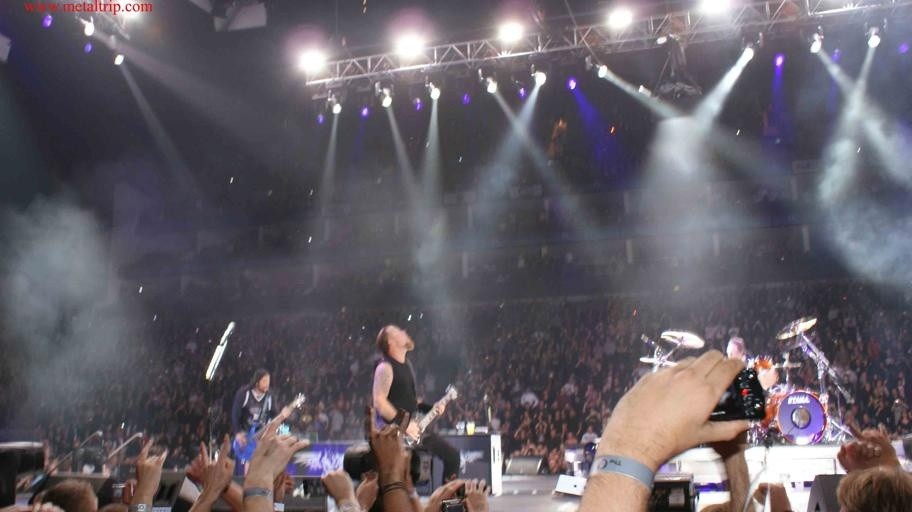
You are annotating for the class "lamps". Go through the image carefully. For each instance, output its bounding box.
[315,4,909,123]
[40,2,130,69]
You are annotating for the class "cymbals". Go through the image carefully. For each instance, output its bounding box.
[641,357,673,366]
[662,331,704,350]
[776,316,817,340]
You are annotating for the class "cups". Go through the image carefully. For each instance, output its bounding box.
[457,422,466,435]
[467,420,475,435]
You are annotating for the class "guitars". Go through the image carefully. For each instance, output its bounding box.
[233,393,306,459]
[402,385,458,449]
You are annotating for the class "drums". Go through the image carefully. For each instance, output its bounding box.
[755,356,779,389]
[762,391,827,445]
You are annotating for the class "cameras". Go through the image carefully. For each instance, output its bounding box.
[456,483,492,499]
[708,368,766,421]
